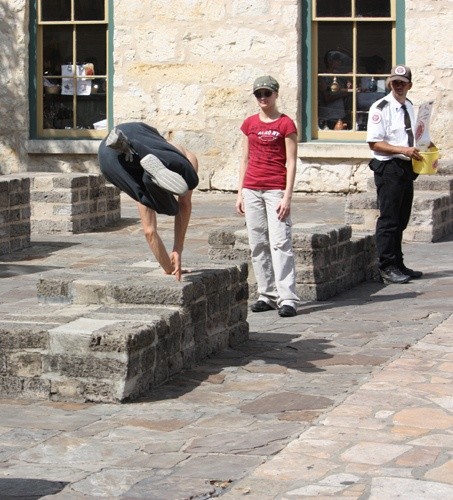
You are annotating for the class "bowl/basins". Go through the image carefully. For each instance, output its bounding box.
[45,85,59,94]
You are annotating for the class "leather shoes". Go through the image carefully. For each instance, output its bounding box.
[380,267,411,284]
[279,305,297,316]
[251,301,274,312]
[399,266,423,279]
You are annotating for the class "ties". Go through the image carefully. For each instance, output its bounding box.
[402,105,413,147]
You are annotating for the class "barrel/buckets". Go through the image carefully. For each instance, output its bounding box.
[412,147,439,175]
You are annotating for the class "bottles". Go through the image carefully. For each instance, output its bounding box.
[369,77,377,92]
[345,79,352,89]
[330,77,339,92]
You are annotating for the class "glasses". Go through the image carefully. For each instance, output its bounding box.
[254,91,277,98]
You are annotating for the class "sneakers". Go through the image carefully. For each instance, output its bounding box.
[105,127,139,162]
[140,154,189,194]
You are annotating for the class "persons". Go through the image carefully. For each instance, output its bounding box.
[366,64,442,286]
[98,123,199,282]
[236,77,298,317]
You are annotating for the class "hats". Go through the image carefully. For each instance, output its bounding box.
[253,75,279,93]
[389,65,411,83]
